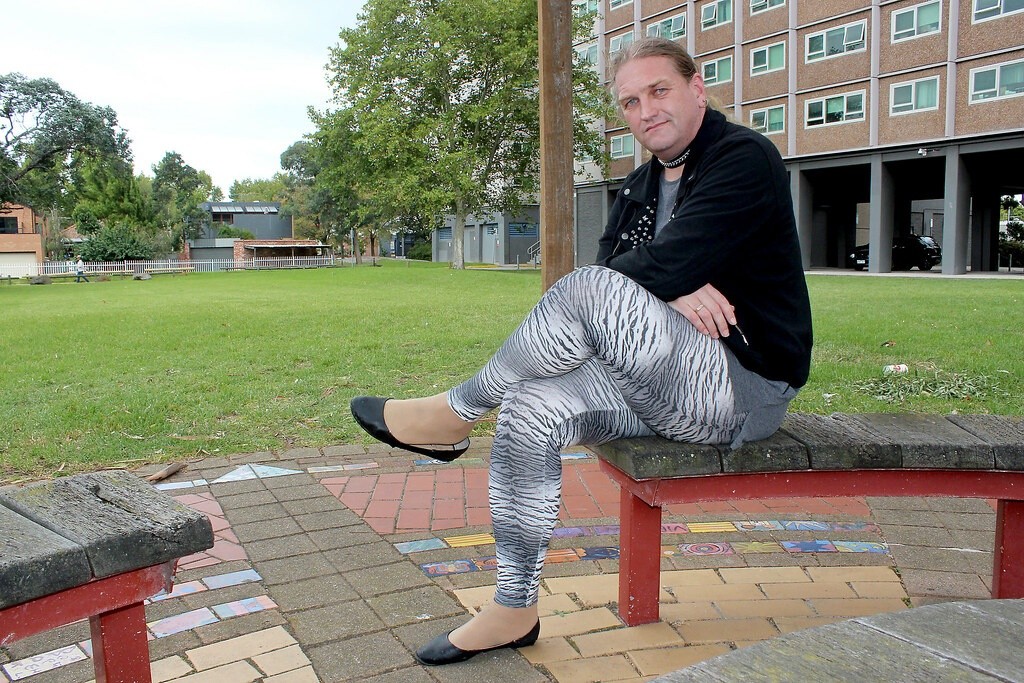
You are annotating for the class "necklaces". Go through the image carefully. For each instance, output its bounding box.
[658,143,691,169]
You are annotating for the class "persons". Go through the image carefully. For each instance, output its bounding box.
[351,38,814,665]
[75,255,89,283]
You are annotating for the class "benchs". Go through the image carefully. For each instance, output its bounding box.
[0,468,214,683]
[583,414,1024,624]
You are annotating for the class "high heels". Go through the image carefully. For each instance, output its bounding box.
[413,610,540,666]
[351,395,470,463]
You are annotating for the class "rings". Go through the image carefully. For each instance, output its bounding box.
[694,304,704,312]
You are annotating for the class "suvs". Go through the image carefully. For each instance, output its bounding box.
[849,235,943,272]
